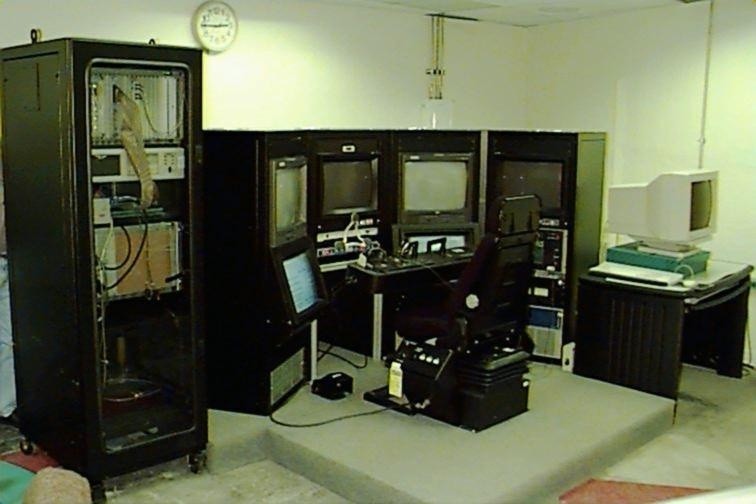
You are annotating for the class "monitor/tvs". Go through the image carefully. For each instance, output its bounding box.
[272,237,330,325]
[494,151,567,218]
[397,152,474,227]
[317,151,383,221]
[269,155,308,248]
[607,169,720,252]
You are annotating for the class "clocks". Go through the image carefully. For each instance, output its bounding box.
[192,2,237,56]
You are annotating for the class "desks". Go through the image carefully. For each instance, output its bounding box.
[573,262,755,422]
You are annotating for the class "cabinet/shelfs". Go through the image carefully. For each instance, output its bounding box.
[1,36,211,504]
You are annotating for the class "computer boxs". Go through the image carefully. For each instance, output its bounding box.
[606,241,710,274]
